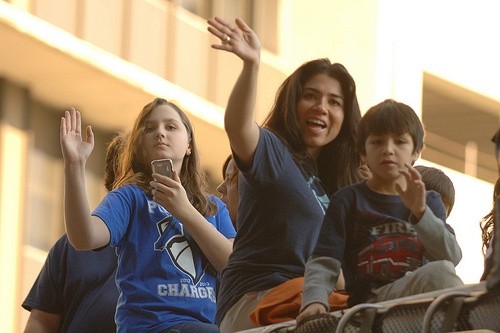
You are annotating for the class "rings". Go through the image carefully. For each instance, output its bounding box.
[222,36,230,44]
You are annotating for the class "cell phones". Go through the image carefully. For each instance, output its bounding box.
[151,159,173,183]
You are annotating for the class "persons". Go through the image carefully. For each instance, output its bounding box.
[60,98,237,333]
[206,15,362,333]
[217,153,237,228]
[20,134,131,333]
[296,95,500,325]
